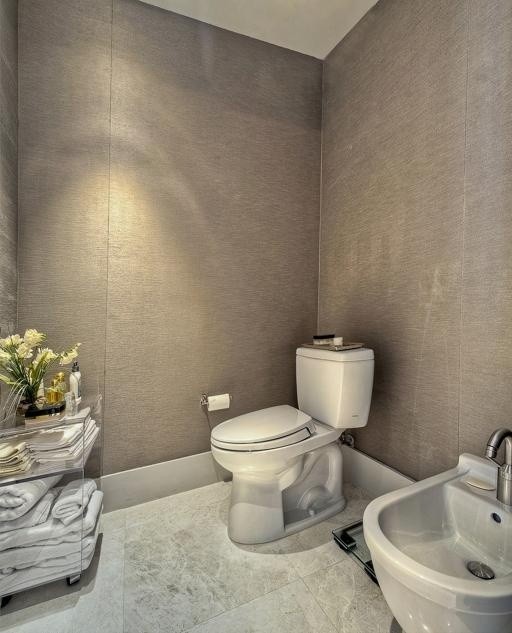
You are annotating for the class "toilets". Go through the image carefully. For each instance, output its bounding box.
[211,338,375,545]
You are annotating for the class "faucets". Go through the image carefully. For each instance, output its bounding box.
[485,429,512,505]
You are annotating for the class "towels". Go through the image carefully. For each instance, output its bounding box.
[1,419,104,601]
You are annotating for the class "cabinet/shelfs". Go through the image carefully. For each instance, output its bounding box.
[0,393,104,611]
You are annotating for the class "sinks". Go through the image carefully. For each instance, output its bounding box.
[363,451,512,633]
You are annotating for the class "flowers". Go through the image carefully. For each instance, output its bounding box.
[0,316,83,431]
